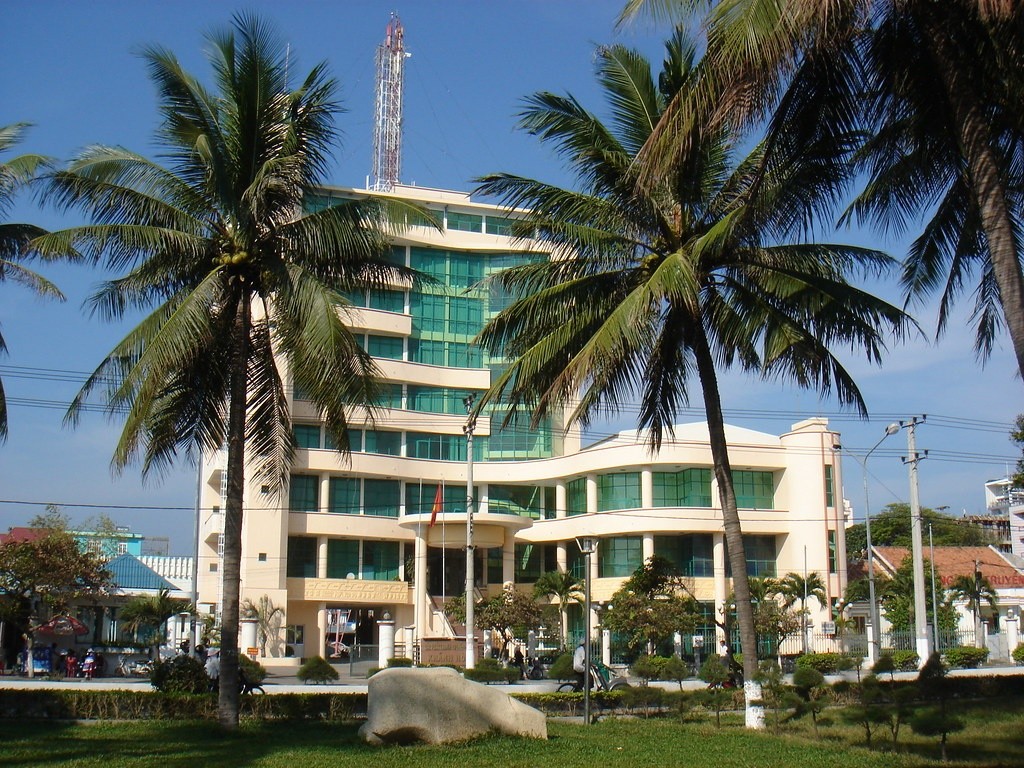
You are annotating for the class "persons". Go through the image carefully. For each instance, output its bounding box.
[514,647,527,677]
[204,648,220,691]
[47,642,64,657]
[574,639,594,691]
[0,640,8,674]
[177,642,189,656]
[720,641,728,657]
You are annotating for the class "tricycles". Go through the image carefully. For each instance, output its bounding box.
[556,660,632,693]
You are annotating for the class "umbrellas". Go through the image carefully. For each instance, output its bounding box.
[38,615,89,635]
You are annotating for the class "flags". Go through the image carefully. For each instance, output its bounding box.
[430,486,440,527]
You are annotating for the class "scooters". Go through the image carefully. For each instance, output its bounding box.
[66,651,99,681]
[114,654,155,679]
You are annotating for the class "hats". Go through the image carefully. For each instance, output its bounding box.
[580,639,585,644]
[207,647,220,656]
[87,648,94,652]
[180,642,188,648]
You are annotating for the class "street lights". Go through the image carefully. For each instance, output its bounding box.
[834,598,853,658]
[861,422,900,667]
[460,389,478,670]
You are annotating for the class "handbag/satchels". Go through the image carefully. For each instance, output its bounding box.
[596,663,610,682]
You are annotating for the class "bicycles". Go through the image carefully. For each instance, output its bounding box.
[211,677,267,696]
[513,658,544,680]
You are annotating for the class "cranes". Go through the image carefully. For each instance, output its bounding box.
[372,12,411,189]
